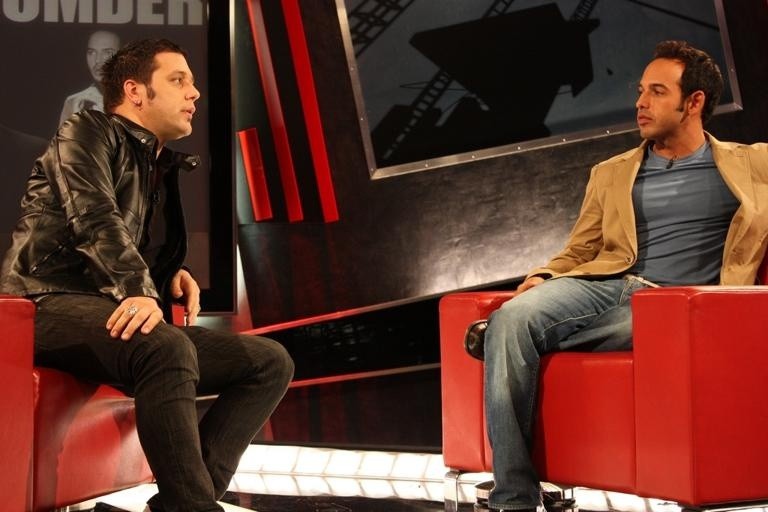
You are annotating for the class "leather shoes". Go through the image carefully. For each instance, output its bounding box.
[463,318,488,361]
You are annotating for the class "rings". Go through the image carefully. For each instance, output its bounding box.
[127,307,138,314]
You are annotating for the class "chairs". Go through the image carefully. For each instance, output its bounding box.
[0,293,186,512]
[437,229,767,509]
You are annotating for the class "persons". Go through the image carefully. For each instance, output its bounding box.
[478,38,768,512]
[0,41,298,512]
[56,26,123,126]
